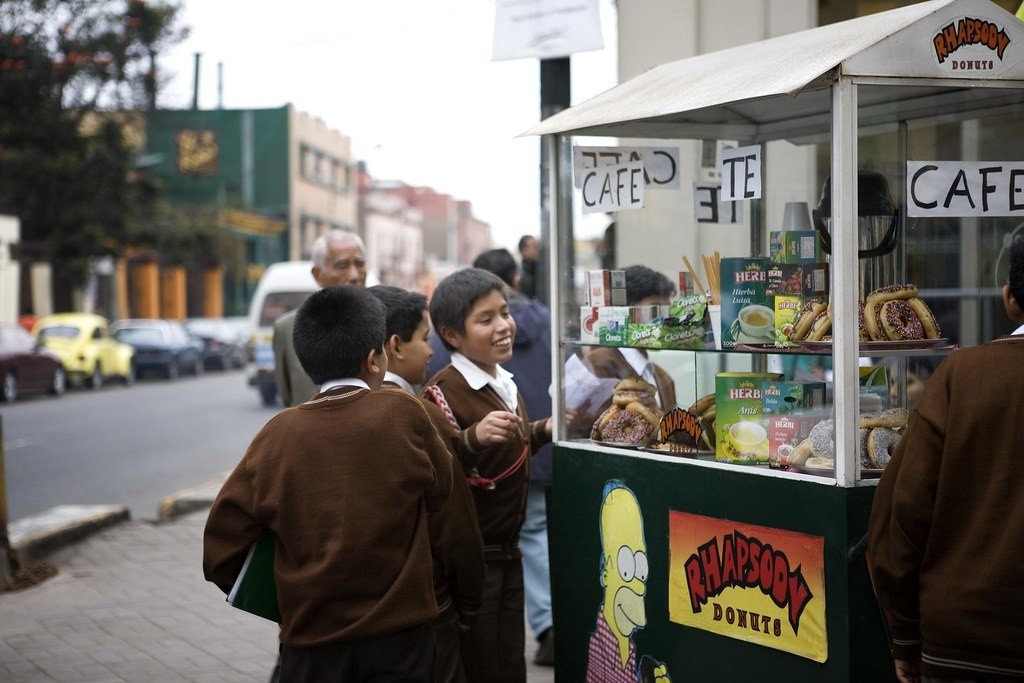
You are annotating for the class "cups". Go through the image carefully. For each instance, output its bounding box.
[781,202,811,231]
[738,304,775,340]
[730,422,767,450]
[708,304,722,350]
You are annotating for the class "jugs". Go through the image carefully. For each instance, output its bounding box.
[811,171,901,297]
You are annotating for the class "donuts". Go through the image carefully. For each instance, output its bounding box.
[787,407,911,469]
[588,378,717,453]
[787,283,942,342]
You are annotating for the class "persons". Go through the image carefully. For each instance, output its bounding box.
[201,287,486,683]
[426,247,556,672]
[547,265,678,443]
[417,265,579,683]
[516,235,542,298]
[271,226,367,408]
[865,237,1024,683]
[366,286,469,683]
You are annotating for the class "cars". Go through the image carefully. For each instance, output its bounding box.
[0,319,65,402]
[108,317,205,379]
[241,258,379,403]
[30,309,137,388]
[185,313,252,369]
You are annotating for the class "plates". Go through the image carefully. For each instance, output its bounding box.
[589,439,640,447]
[792,337,950,349]
[721,433,769,461]
[796,466,885,479]
[730,318,775,343]
[646,448,715,455]
